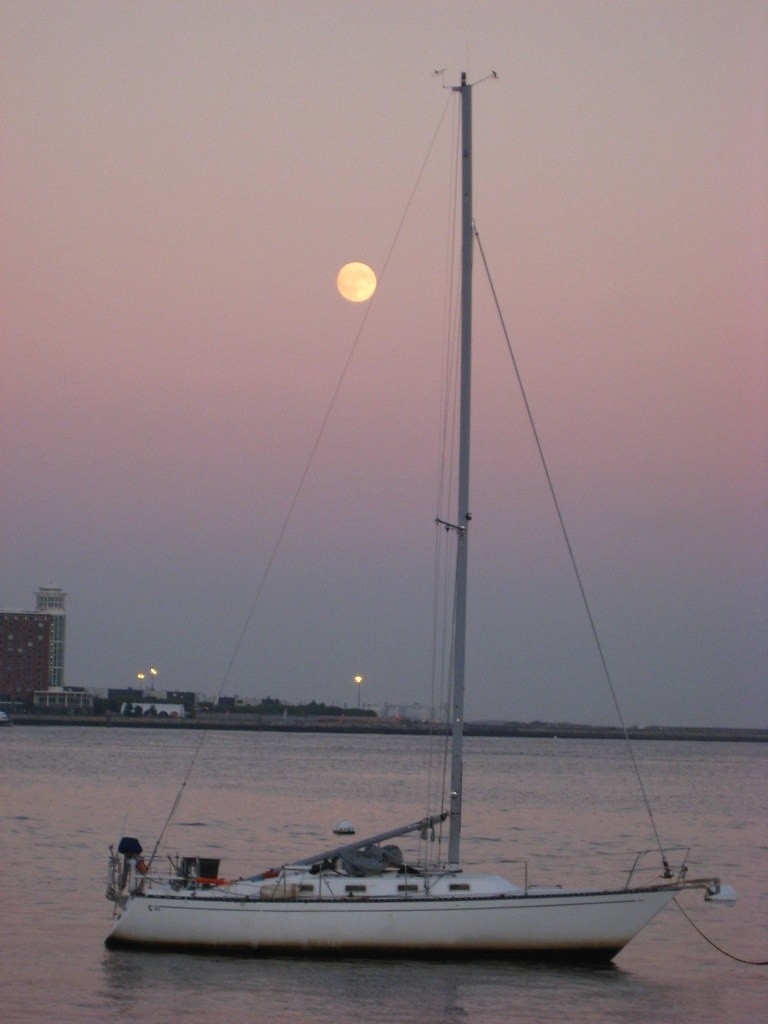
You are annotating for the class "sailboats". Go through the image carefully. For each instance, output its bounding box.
[92,69,722,960]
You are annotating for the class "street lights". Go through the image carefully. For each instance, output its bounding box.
[136,673,145,690]
[150,668,157,690]
[355,675,362,709]
